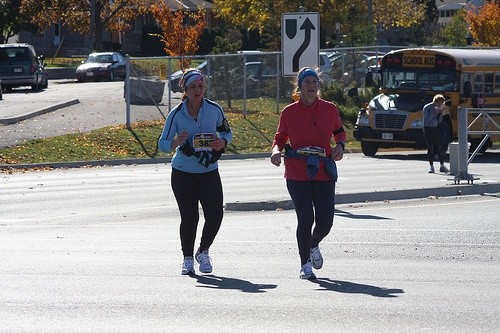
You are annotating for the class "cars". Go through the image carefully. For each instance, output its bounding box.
[0,43,48,92]
[76,52,132,82]
[168,51,386,100]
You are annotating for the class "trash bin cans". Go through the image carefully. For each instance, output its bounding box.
[450,142,471,174]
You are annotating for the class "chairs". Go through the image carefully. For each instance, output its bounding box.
[0,50,12,63]
[15,51,25,61]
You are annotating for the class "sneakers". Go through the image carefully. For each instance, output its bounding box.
[195,247,212,273]
[182,256,195,275]
[310,244,323,269]
[300,258,316,279]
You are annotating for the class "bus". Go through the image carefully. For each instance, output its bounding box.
[353,47,500,158]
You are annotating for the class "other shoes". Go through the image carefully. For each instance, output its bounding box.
[440,166,449,173]
[429,167,434,173]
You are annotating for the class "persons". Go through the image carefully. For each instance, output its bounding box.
[422,94,449,173]
[157,68,232,274]
[270,68,347,279]
